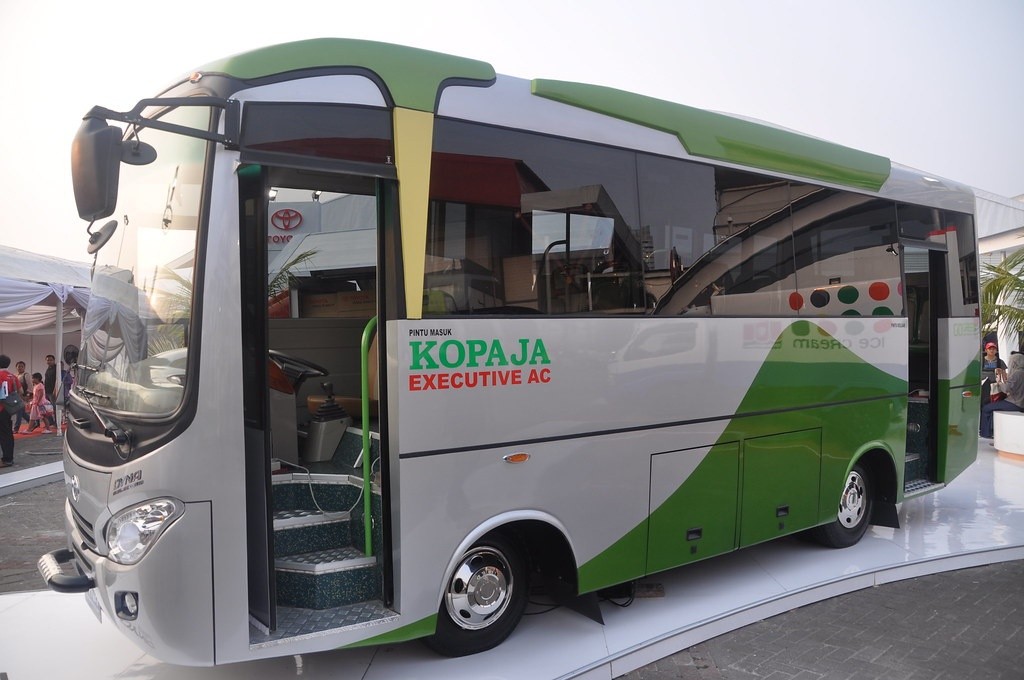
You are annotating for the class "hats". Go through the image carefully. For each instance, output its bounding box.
[985,342,997,349]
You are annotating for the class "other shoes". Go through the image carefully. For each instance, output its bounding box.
[0,458,14,468]
[21,430,31,435]
[41,430,52,434]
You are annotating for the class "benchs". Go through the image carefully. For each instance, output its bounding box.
[993,411,1024,462]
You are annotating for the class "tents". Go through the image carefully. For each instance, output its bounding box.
[0,245,139,437]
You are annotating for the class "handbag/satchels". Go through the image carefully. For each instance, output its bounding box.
[2,391,25,416]
[21,373,29,393]
[990,383,1007,403]
[37,403,54,416]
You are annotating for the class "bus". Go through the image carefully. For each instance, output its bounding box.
[34,37,980,664]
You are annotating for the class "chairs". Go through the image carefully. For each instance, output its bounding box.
[907,285,929,353]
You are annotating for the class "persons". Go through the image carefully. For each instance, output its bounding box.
[981,343,1008,404]
[0,354,23,467]
[43,355,56,428]
[979,353,1024,438]
[20,373,53,434]
[12,361,38,434]
[61,361,73,399]
